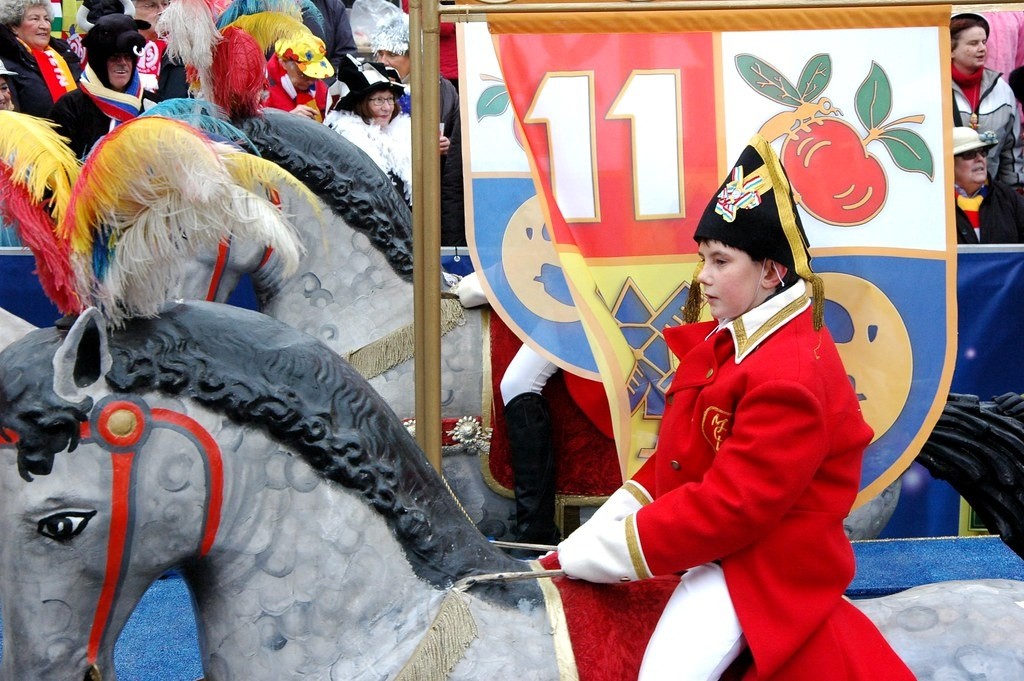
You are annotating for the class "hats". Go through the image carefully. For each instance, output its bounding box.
[953,126,998,156]
[81,13,151,88]
[0,59,19,75]
[232,11,335,79]
[683,134,826,331]
[338,53,390,103]
[950,12,990,38]
[214,25,269,115]
[385,66,406,99]
[350,0,410,53]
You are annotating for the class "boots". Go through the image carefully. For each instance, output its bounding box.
[496,392,562,556]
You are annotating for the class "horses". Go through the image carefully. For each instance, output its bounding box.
[96,105,1020,551]
[0,299,1020,681]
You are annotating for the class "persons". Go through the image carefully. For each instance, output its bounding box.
[558,133,917,681]
[949,12,1024,244]
[0,0,469,250]
[457,268,562,554]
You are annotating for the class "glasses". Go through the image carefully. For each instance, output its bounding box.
[107,53,134,63]
[136,2,170,10]
[956,149,989,160]
[368,95,396,106]
